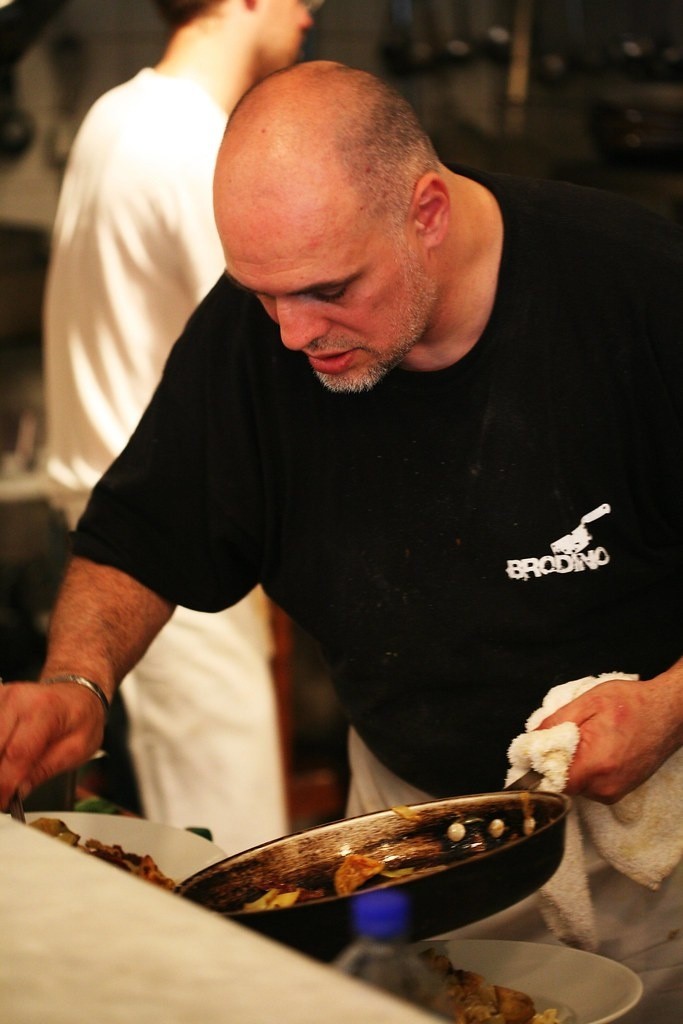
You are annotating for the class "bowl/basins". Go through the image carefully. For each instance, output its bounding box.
[3,811,228,885]
[403,940,643,1024]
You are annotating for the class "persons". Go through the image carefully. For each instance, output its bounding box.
[0,59,683,1023]
[42,0,316,858]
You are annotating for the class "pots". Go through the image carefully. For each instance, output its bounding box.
[172,770,572,966]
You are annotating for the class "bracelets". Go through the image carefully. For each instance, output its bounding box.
[42,676,110,723]
